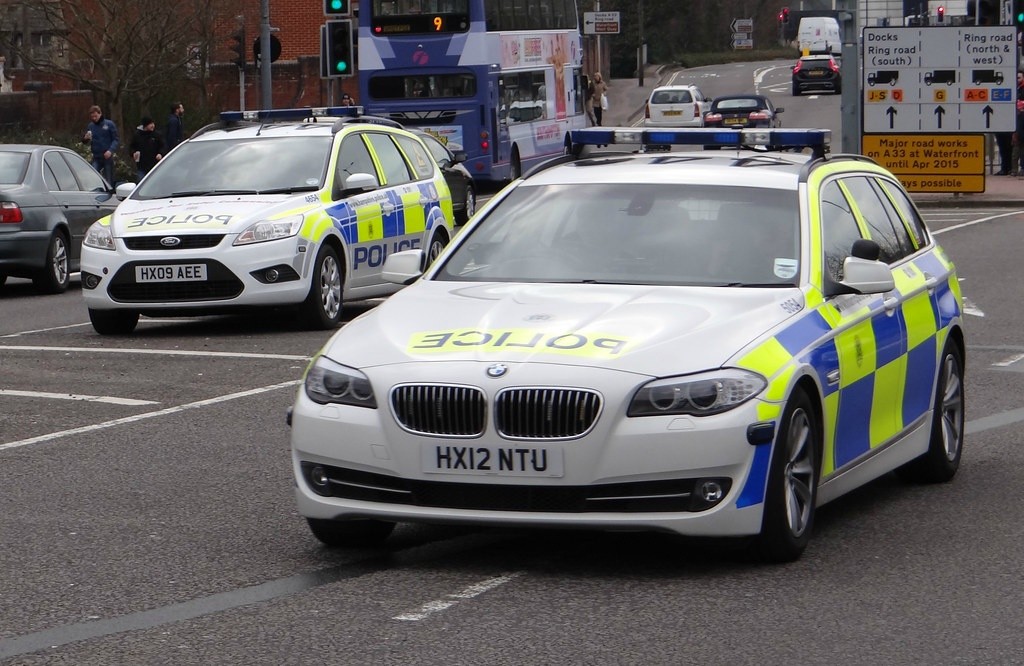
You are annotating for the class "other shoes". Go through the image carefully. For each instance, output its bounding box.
[994,169,1008,175]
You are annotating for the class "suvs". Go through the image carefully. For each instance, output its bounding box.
[645,82,712,128]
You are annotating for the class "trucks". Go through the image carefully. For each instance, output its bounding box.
[796,16,841,55]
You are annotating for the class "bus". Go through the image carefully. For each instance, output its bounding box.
[354,0,586,194]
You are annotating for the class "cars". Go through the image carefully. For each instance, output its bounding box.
[79,106,476,336]
[703,94,784,150]
[285,128,966,564]
[790,55,841,97]
[0,143,128,294]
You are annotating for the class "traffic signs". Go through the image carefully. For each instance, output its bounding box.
[862,25,1017,134]
[583,11,620,35]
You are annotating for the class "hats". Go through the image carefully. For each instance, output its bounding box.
[343,94,350,99]
[142,116,154,128]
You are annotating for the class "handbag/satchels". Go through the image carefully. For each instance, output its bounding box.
[600,93,608,110]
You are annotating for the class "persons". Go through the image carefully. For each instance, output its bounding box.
[167,102,184,153]
[584,72,608,126]
[81,105,120,189]
[340,93,357,106]
[993,71,1024,177]
[130,116,164,182]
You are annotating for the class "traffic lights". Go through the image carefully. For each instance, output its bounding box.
[1011,0,1024,31]
[778,8,789,23]
[323,0,351,17]
[326,19,353,80]
[231,28,245,70]
[938,7,943,22]
